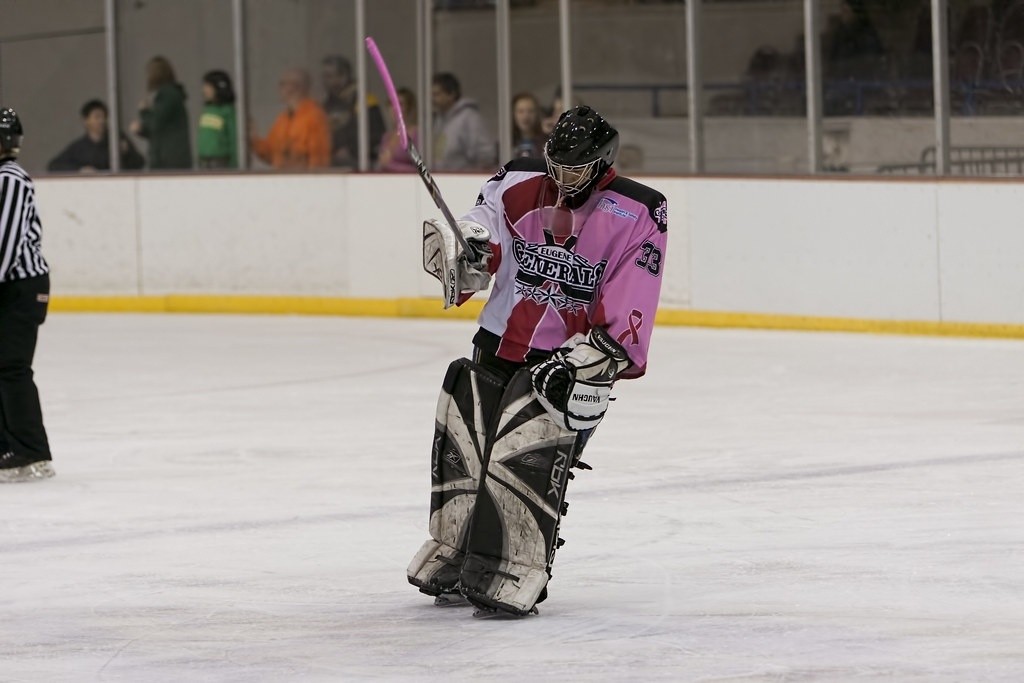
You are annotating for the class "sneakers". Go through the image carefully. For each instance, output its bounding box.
[0,450,57,482]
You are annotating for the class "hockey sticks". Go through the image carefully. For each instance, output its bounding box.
[363,33,487,273]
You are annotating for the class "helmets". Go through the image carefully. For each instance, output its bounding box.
[543,105,620,209]
[0,108,22,133]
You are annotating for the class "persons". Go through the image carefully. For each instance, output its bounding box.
[406,105,668,618]
[248,54,419,173]
[0,109,52,469]
[49,55,238,174]
[431,71,577,171]
